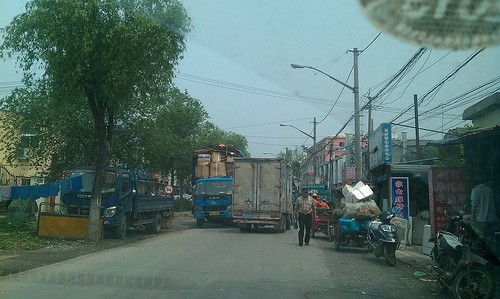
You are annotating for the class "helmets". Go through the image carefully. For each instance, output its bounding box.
[311,193,318,197]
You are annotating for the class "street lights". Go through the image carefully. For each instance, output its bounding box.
[279,123,316,186]
[291,63,363,183]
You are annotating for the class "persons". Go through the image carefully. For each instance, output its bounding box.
[319,199,328,208]
[312,192,320,204]
[294,187,319,247]
[470,178,500,231]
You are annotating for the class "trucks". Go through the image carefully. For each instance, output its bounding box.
[61,166,175,240]
[231,157,294,234]
[190,144,246,228]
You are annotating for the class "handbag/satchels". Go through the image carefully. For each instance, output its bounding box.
[313,220,319,232]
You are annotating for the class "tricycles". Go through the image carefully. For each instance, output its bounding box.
[310,199,372,250]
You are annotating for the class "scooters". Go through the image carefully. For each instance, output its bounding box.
[363,210,401,266]
[427,208,499,299]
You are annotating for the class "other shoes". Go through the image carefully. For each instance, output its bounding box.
[299,242,303,247]
[305,243,309,245]
